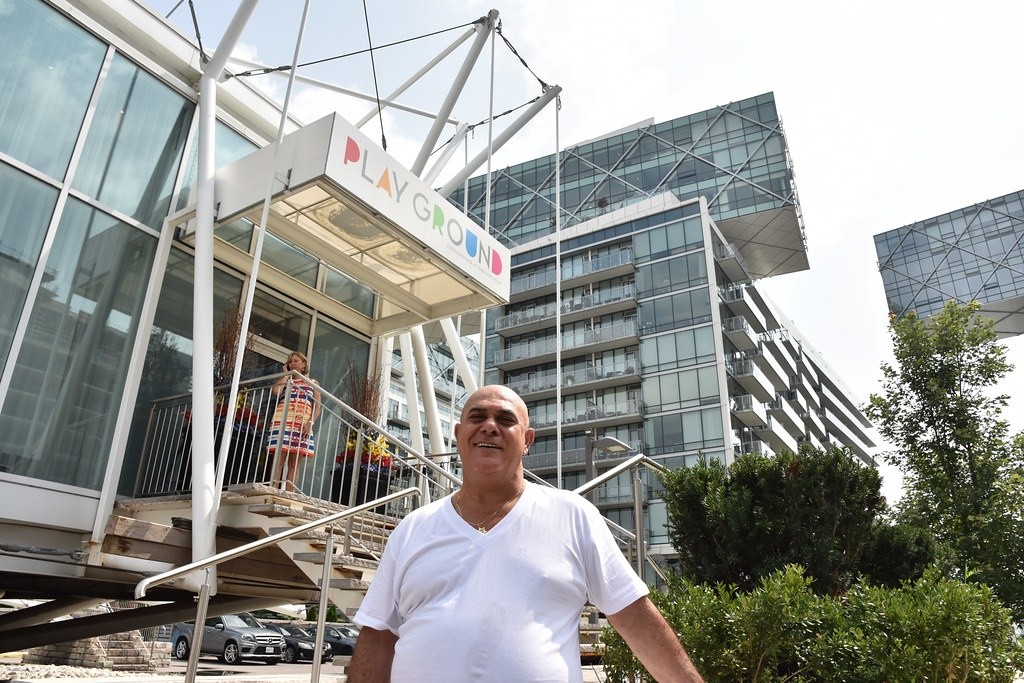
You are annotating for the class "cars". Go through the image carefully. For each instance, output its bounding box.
[170,615,287,666]
[262,622,332,664]
[305,626,357,656]
[330,627,360,638]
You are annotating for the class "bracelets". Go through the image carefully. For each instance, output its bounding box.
[309,421,314,425]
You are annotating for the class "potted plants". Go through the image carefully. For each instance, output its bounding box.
[565,375,575,386]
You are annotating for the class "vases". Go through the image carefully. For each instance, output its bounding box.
[178,432,258,493]
[328,468,395,515]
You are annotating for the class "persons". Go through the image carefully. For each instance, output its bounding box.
[266,352,321,493]
[346,384,704,683]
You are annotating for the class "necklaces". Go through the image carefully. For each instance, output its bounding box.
[456,491,520,534]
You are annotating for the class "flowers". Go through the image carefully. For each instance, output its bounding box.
[334,356,400,476]
[176,298,277,435]
